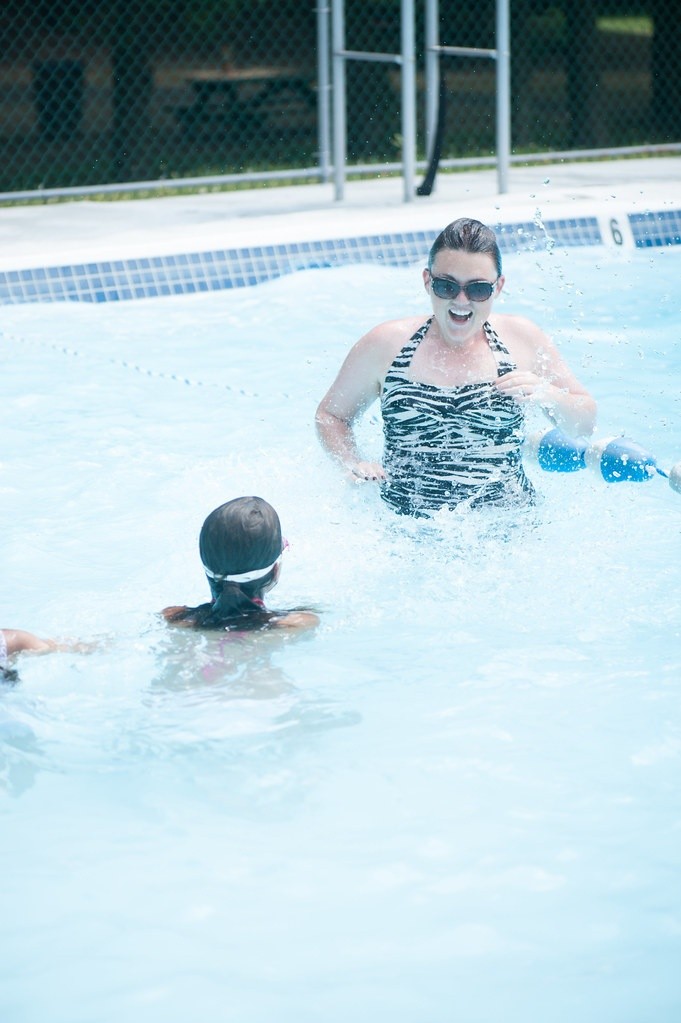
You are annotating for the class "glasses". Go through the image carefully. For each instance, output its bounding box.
[280,536,291,556]
[430,275,498,302]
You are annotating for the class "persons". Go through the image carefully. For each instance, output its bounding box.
[0,492,318,652]
[313,218,593,515]
